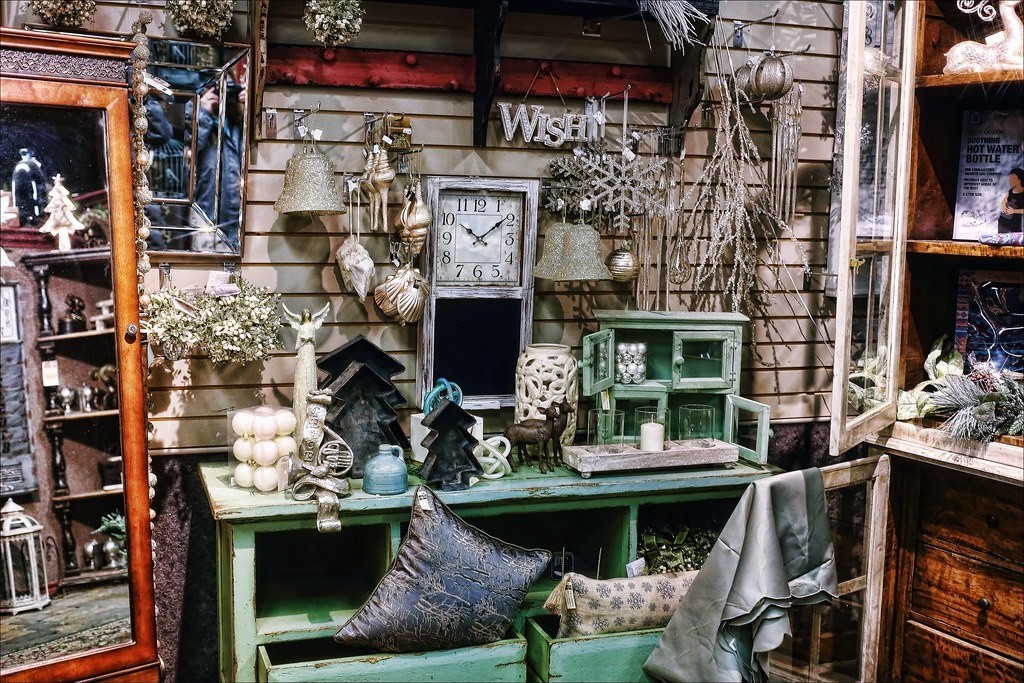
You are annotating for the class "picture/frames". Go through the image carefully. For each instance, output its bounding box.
[20,21,254,264]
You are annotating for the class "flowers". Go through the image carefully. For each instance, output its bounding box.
[165,0,235,42]
[137,275,287,365]
[20,0,97,28]
[302,0,367,50]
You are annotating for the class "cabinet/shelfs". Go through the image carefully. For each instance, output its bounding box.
[582,310,770,466]
[752,0,1024,683]
[22,247,130,590]
[198,439,787,683]
[0,48,161,683]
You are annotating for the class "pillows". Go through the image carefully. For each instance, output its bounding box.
[334,480,555,653]
[544,571,701,640]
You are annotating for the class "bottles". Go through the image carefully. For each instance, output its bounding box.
[12,147,47,229]
[362,444,407,495]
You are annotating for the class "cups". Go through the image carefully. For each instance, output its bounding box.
[587,409,625,455]
[679,404,714,448]
[634,406,672,452]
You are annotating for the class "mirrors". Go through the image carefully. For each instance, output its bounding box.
[0,102,140,675]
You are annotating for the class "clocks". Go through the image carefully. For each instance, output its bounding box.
[425,175,540,299]
